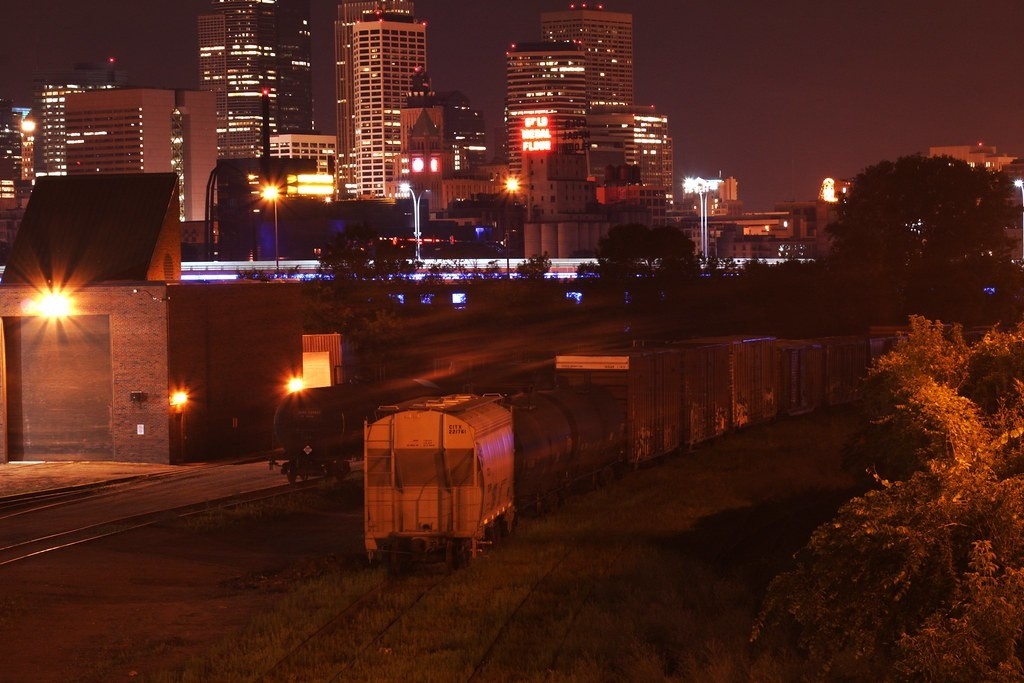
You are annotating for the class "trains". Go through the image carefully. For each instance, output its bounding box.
[363,320,1005,575]
[272,368,388,491]
